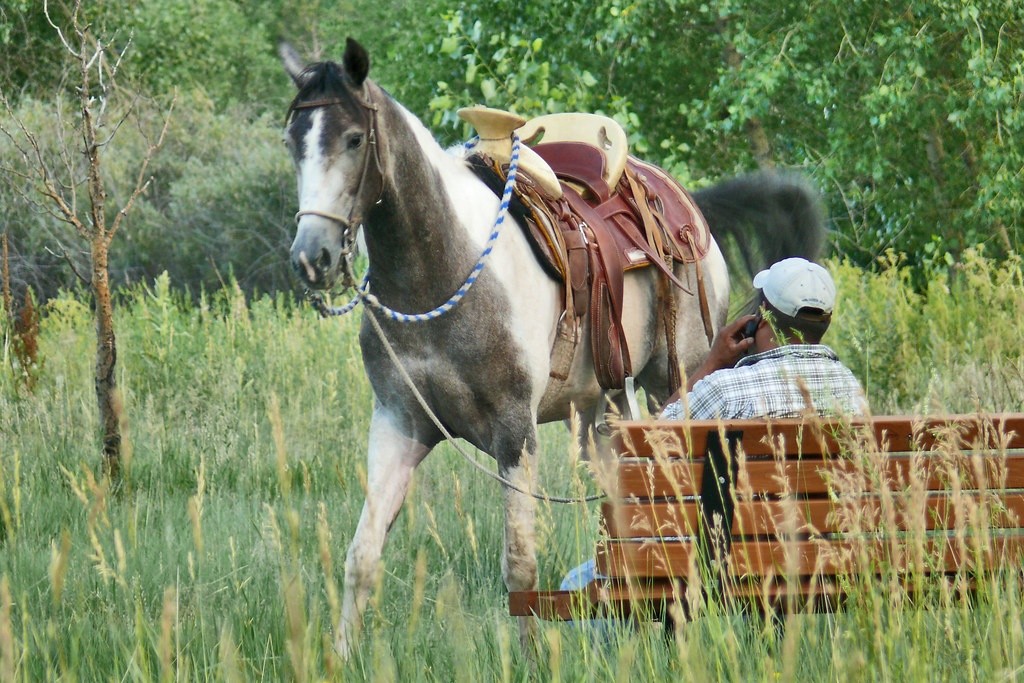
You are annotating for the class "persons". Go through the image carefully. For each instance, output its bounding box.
[558,257,874,592]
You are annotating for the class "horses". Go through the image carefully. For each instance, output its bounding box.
[277,36,825,660]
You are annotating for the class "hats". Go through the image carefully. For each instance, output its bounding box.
[753,257,836,320]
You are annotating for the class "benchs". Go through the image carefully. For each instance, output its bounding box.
[508,413,1024,648]
